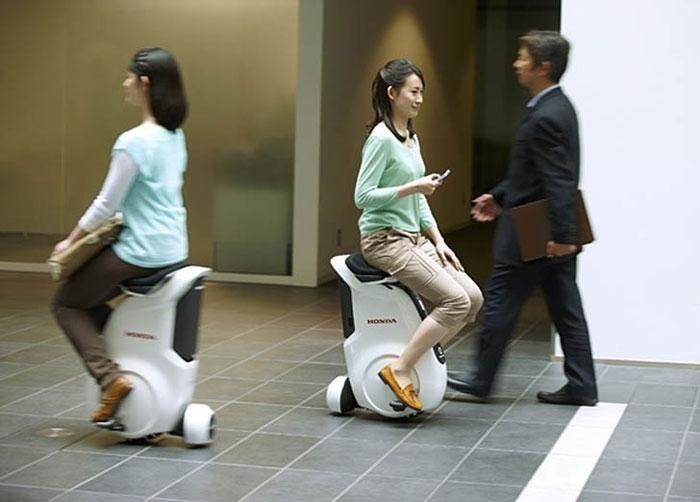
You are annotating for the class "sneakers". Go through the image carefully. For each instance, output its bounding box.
[377,363,424,413]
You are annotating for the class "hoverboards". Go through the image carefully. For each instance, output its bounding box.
[326,254,448,423]
[91,265,215,449]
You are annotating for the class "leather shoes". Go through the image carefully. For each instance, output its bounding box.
[536,387,599,406]
[447,375,489,399]
[88,376,133,422]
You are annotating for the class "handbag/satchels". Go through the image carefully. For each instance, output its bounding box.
[45,216,125,284]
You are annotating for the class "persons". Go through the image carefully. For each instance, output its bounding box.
[353,57,484,411]
[447,28,599,406]
[49,47,190,424]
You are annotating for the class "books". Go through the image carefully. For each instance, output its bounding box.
[510,189,594,264]
